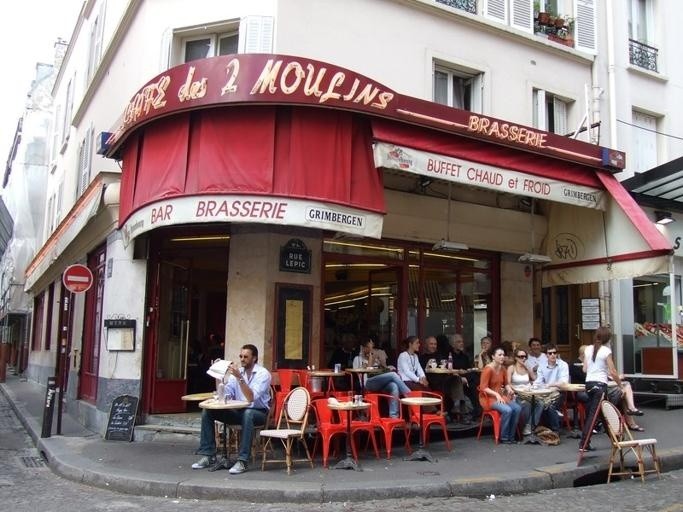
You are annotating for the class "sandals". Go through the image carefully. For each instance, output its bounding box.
[628,423,638,430]
[625,406,643,417]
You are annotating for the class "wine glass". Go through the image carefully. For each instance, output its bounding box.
[442,359,447,367]
[212,391,219,401]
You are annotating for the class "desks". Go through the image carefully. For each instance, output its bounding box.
[556,382,586,432]
[425,367,481,424]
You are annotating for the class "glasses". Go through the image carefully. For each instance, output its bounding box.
[238,354,247,359]
[547,351,556,355]
[516,355,526,359]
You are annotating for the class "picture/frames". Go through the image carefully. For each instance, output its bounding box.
[272,282,314,371]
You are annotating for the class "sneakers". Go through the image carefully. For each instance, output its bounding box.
[501,424,532,444]
[228,461,248,475]
[579,443,594,452]
[191,456,217,469]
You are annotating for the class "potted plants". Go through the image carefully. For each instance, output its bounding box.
[534,1,575,39]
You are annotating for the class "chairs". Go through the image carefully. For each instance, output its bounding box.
[180,368,452,475]
[476,385,521,446]
[600,400,662,484]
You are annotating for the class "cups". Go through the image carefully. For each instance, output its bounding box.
[334,364,342,373]
[224,394,232,405]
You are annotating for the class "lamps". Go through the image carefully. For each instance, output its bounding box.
[654,210,675,225]
[518,197,552,263]
[432,181,469,254]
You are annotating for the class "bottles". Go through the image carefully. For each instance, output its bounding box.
[368,352,374,367]
[355,395,362,405]
[432,359,437,369]
[448,355,453,371]
[358,345,365,369]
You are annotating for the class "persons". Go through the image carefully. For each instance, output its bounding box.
[419,327,645,453]
[192,344,272,474]
[327,334,361,395]
[353,335,412,428]
[397,336,449,416]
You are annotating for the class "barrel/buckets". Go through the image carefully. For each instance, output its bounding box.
[307,376,325,393]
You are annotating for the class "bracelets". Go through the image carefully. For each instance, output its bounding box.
[369,352,374,355]
[239,379,245,385]
